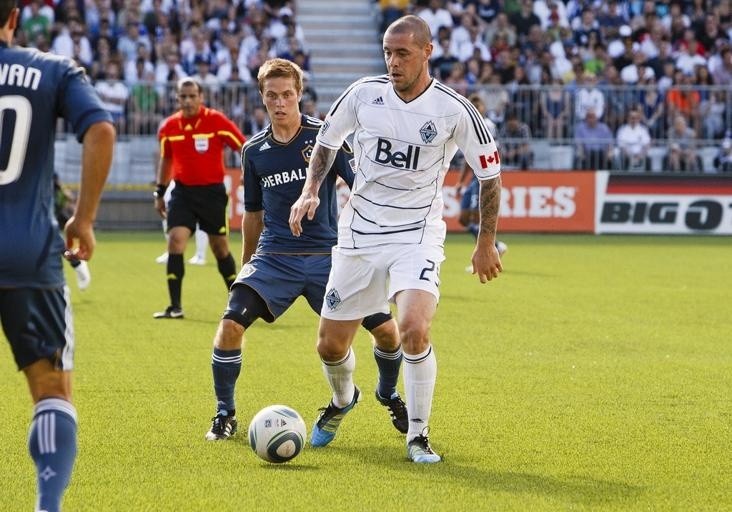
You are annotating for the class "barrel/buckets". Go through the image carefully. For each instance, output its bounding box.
[531,160,551,172]
[52,135,161,183]
[550,144,572,170]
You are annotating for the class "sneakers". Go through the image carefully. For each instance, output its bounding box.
[376,388,409,433]
[310,385,361,447]
[155,252,168,263]
[188,256,205,264]
[153,306,183,318]
[495,241,507,256]
[205,413,237,441]
[75,260,90,289]
[407,434,440,463]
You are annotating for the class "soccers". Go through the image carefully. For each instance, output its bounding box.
[249,404,307,463]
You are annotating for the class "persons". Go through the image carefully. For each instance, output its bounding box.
[371,1,731,146]
[14,0,323,136]
[571,110,651,170]
[455,96,507,271]
[288,15,504,463]
[153,79,248,318]
[719,132,732,170]
[1,0,114,510]
[206,58,409,440]
[667,116,698,169]
[54,172,76,229]
[500,116,533,169]
